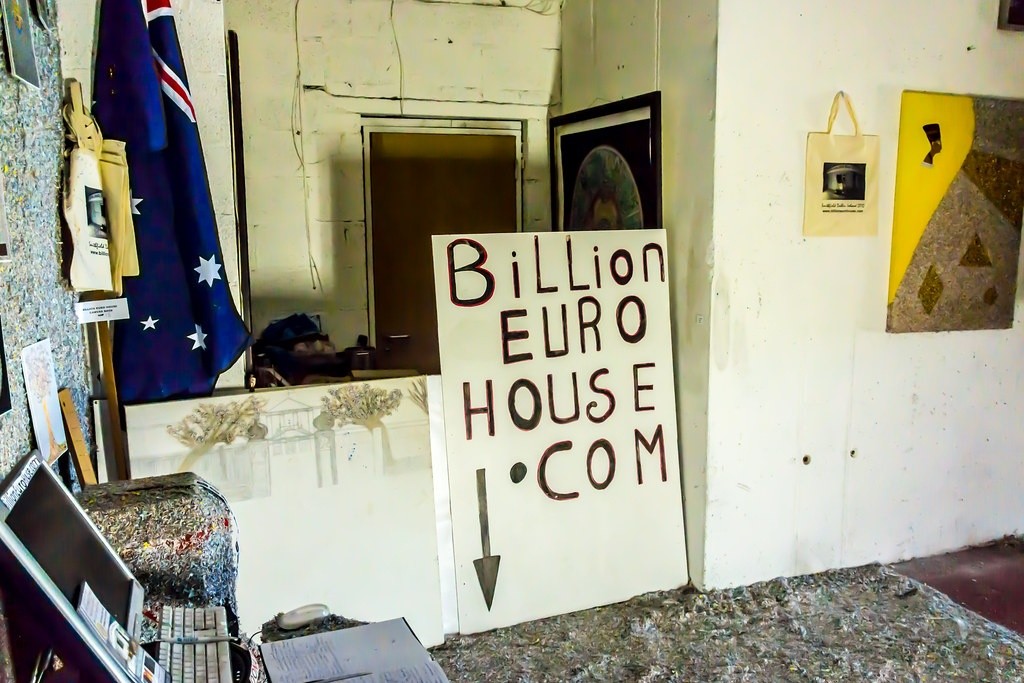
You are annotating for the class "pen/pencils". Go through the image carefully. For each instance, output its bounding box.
[156,633,242,645]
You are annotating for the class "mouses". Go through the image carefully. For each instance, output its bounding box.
[277,603,329,630]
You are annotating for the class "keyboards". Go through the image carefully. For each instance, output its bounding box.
[157,605,232,683]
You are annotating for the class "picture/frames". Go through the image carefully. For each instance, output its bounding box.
[546,88,662,233]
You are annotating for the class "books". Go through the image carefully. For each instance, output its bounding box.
[258,617,449,683]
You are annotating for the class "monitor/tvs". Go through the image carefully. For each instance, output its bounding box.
[1,449,145,683]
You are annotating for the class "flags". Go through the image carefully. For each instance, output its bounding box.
[93,0,253,430]
